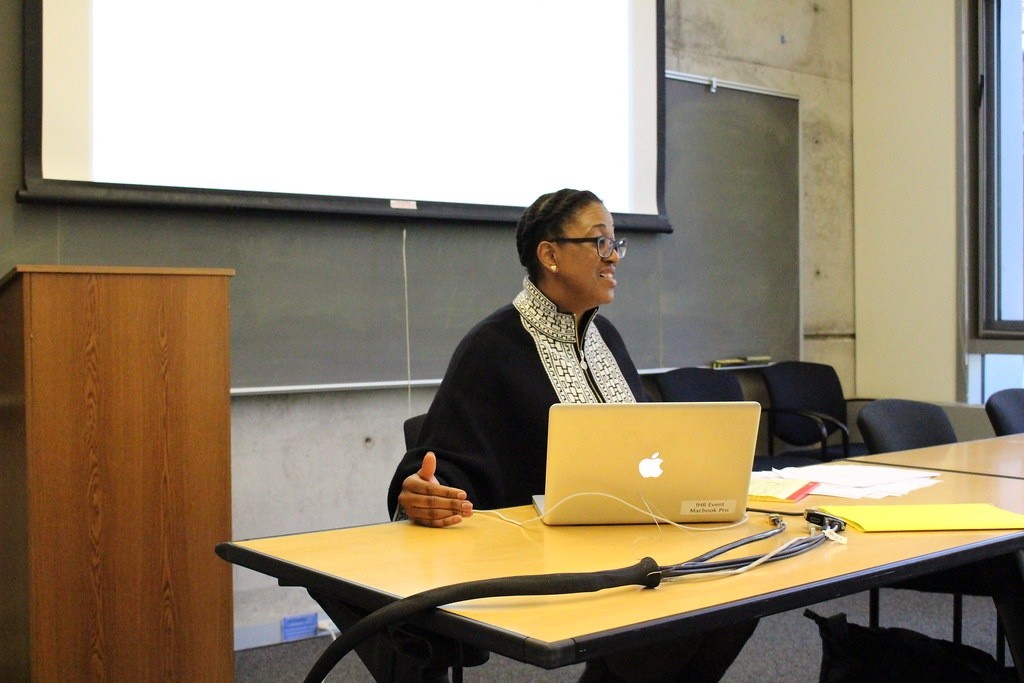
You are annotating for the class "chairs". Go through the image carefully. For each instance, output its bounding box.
[653,367,837,471]
[985,388,1024,438]
[761,360,878,458]
[854,397,1009,663]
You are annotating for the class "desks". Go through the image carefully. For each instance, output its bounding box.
[213,460,1024,683]
[844,433,1024,481]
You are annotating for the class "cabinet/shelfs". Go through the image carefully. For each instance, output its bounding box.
[0,263,237,683]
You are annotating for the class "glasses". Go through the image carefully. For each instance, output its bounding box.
[547,236,629,260]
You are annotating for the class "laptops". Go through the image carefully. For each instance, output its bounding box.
[531,401,762,528]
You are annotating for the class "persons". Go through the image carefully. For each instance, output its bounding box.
[386,187,767,683]
